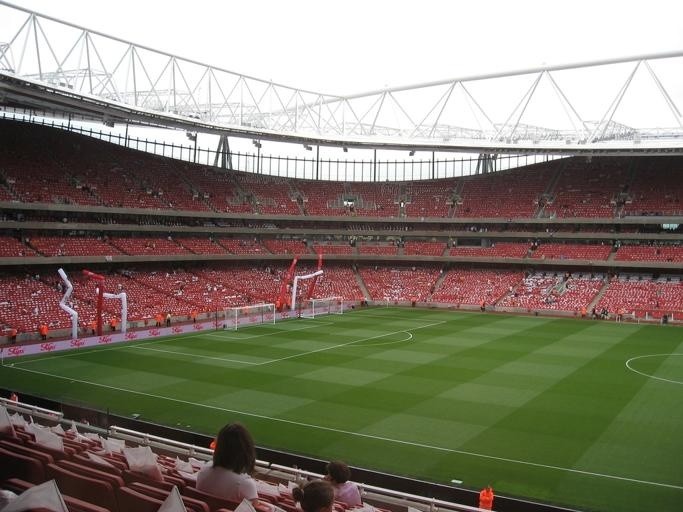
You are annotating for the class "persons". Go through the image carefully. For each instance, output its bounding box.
[0,115,683,239]
[1,239,682,337]
[40,323,48,340]
[291,465,298,475]
[79,417,90,426]
[195,421,259,501]
[10,327,17,345]
[8,391,18,408]
[479,484,495,511]
[209,437,217,451]
[293,480,335,512]
[325,459,362,505]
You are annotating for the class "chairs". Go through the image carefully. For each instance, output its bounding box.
[1,417,394,512]
[2,120,682,340]
